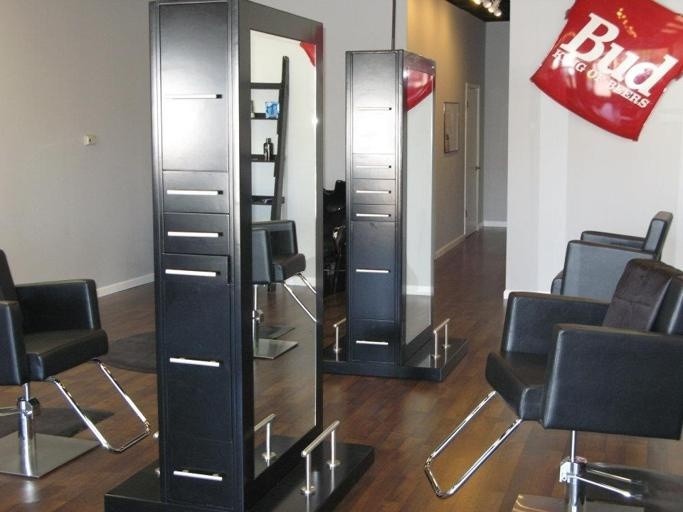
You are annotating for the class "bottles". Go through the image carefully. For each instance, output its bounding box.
[264,137,273,161]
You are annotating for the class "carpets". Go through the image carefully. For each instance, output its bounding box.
[90,330,157,374]
[0,405,114,440]
[553,460,683,512]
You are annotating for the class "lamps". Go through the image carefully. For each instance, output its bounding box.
[473,0,502,17]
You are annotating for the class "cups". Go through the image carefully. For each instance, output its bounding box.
[266,102,278,118]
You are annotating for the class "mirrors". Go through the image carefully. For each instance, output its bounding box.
[403,68,434,347]
[249,27,318,479]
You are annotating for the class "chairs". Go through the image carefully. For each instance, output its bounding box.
[551,209,673,299]
[422,255,683,512]
[251,219,316,360]
[0,249,151,480]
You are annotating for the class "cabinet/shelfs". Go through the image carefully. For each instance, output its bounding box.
[249,55,290,293]
[323,48,470,382]
[105,0,374,512]
